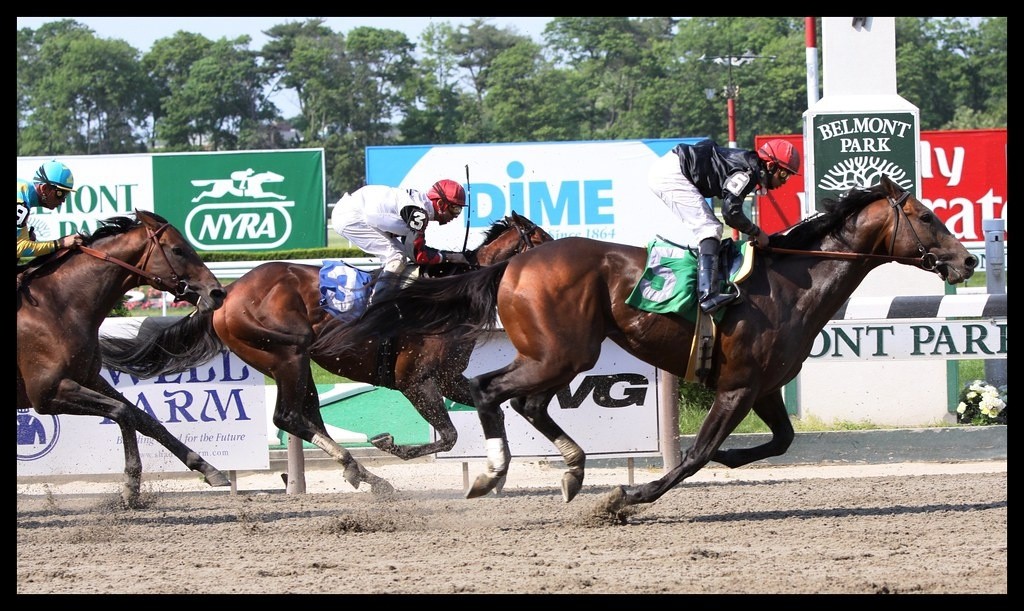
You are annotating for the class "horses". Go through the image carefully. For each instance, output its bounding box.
[98,209,554,503]
[13,209,231,513]
[310,169,979,522]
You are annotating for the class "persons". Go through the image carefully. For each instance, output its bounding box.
[648,139,802,315]
[330,179,469,311]
[17,160,83,262]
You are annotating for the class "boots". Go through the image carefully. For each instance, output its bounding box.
[366,270,402,308]
[697,253,737,315]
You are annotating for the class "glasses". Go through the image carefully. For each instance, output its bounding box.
[777,168,790,183]
[433,181,462,218]
[36,167,68,200]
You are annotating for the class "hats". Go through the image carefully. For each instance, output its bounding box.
[757,139,802,176]
[427,180,469,207]
[32,159,78,193]
